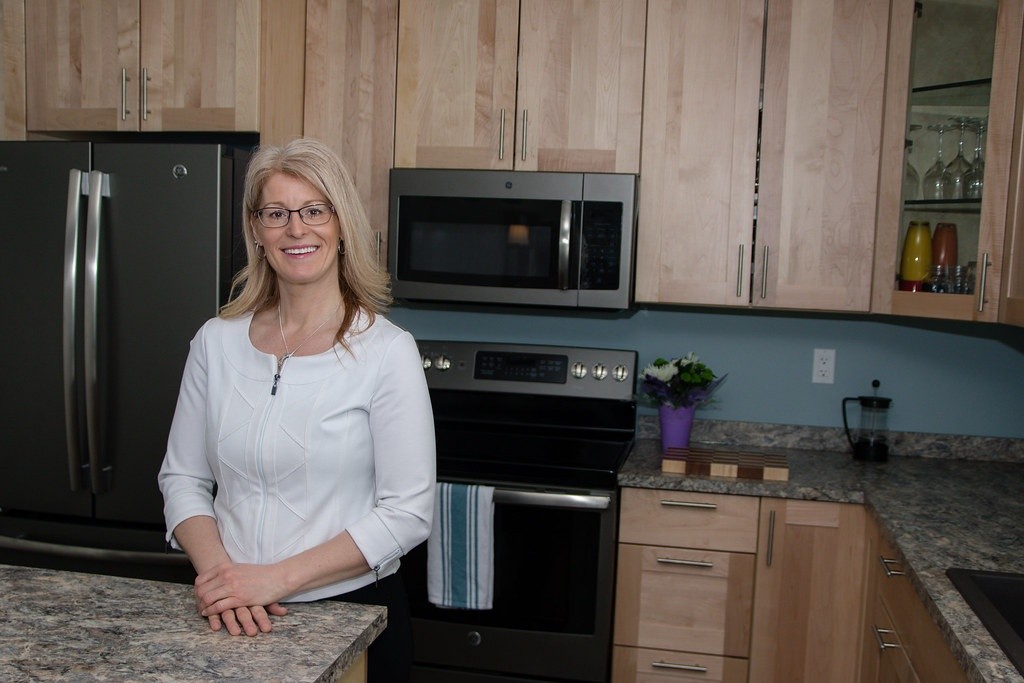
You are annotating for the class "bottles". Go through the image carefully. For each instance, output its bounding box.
[899,220,974,295]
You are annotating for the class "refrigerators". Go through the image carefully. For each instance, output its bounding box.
[1,138,251,585]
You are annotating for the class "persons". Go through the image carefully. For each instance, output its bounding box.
[158,138,436,683]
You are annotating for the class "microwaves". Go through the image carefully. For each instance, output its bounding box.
[388,169,639,311]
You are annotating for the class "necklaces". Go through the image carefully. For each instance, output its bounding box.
[274,294,342,371]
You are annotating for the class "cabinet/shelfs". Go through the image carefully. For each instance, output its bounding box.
[394,0,647,176]
[25,0,305,148]
[304,1,399,274]
[611,488,760,683]
[864,517,967,683]
[748,497,867,683]
[869,1,1024,326]
[635,0,889,316]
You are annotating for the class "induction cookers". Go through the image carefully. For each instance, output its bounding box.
[415,338,640,489]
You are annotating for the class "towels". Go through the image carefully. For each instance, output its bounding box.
[426,481,495,610]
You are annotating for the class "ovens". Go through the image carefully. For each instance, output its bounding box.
[395,483,620,683]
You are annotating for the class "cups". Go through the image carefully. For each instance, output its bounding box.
[842,395,893,463]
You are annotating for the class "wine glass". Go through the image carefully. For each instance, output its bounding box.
[904,116,988,198]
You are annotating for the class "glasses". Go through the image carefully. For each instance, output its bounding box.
[252,203,335,229]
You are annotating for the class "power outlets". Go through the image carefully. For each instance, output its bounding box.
[811,349,836,383]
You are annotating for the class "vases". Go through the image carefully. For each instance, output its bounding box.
[660,405,694,450]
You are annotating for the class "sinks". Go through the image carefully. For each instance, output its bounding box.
[944,567,1024,680]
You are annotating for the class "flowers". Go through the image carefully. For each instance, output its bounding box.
[639,352,729,410]
